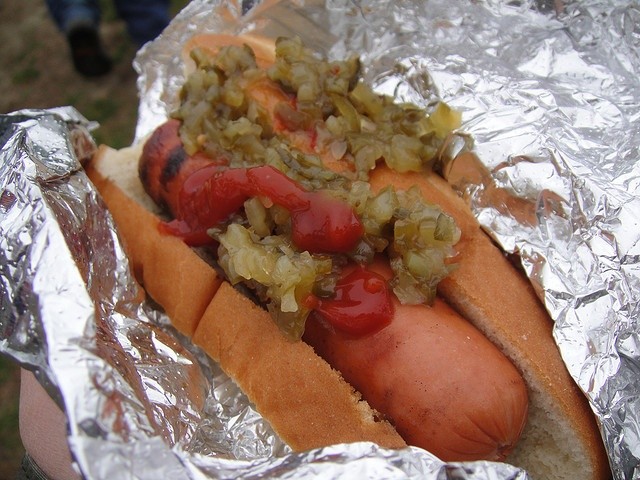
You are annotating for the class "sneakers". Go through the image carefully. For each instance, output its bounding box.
[65,7,112,80]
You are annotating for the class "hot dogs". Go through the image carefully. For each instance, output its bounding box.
[65,36,615,479]
